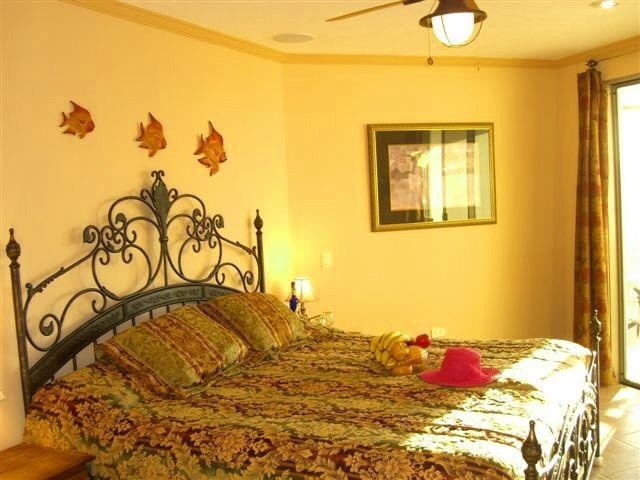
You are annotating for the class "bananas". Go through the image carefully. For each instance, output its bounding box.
[370,330,410,366]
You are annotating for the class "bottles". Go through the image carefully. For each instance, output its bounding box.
[290,282,300,317]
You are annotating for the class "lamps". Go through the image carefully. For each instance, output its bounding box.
[417,0,488,49]
[286,279,317,322]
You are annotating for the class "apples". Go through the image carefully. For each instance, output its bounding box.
[416,334,431,348]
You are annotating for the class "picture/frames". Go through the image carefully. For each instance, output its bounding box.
[367,123,500,233]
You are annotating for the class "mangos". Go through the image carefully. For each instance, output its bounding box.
[391,365,413,375]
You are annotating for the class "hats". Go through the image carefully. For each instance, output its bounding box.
[419,345,501,386]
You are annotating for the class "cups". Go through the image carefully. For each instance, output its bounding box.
[319,312,334,328]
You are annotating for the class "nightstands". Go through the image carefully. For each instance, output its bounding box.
[0,445,100,480]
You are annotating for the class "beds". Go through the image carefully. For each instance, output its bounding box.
[0,169,603,480]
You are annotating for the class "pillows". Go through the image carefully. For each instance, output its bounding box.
[97,305,250,396]
[209,293,313,354]
